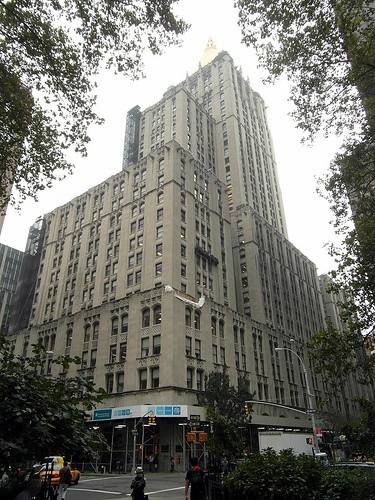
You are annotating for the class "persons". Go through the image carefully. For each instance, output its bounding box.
[149,455,160,473]
[184,457,208,500]
[29,468,36,480]
[15,479,42,500]
[59,461,72,500]
[130,467,146,500]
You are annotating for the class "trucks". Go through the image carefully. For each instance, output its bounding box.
[258,432,314,456]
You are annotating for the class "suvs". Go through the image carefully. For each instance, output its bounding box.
[32,456,63,473]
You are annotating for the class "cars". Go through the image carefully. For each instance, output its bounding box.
[36,464,81,485]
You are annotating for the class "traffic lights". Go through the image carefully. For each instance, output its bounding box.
[152,415,157,423]
[148,415,151,423]
[305,437,312,445]
[244,405,248,415]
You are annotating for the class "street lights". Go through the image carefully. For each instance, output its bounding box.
[275,339,320,453]
